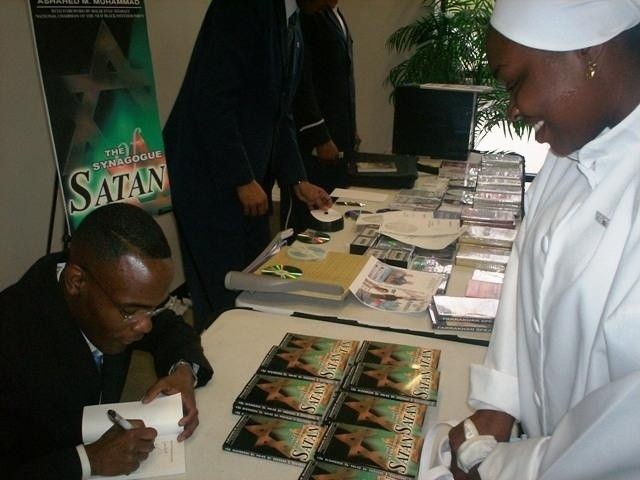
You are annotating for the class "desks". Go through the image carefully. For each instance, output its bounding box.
[233,147,528,345]
[115,302,527,480]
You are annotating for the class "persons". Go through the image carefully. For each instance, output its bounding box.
[448,0,640,479]
[283,0,359,194]
[1,202,214,480]
[164,2,331,320]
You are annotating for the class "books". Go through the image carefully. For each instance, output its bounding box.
[82,392,186,478]
[389,153,525,336]
[356,161,397,174]
[222,332,442,479]
[420,81,493,94]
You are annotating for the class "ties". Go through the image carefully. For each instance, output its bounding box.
[286,11,301,89]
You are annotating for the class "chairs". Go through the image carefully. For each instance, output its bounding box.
[390,82,479,160]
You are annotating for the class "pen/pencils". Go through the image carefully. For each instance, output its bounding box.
[107,409,156,449]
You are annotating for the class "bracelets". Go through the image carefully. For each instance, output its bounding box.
[288,178,306,187]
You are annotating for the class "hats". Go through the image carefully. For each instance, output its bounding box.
[489,0,640,52]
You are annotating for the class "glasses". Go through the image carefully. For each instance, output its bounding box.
[81,264,177,323]
